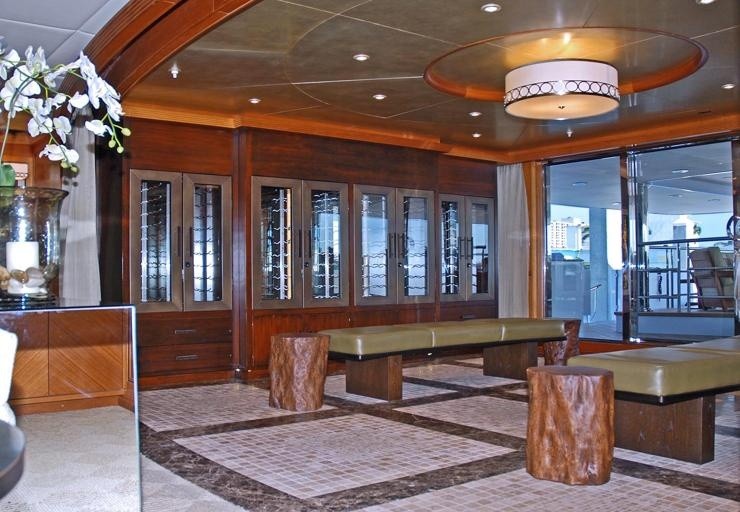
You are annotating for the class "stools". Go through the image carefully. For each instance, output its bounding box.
[525,364,617,488]
[542,316,584,366]
[268,333,330,412]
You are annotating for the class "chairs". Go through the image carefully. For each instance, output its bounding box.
[689,247,735,312]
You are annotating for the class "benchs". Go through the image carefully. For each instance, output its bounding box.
[573,335,740,466]
[317,317,566,398]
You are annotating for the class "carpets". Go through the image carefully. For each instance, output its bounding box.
[141,452,250,511]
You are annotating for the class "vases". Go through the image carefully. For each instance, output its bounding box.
[0,187,69,311]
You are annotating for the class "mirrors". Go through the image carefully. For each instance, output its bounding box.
[1,307,140,509]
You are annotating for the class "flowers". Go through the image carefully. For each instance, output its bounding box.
[0,36,131,186]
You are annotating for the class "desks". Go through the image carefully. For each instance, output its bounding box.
[0,418,27,501]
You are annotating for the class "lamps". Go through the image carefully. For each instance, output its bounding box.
[2,162,29,181]
[505,60,621,122]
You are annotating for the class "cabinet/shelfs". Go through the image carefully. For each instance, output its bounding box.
[353,185,436,329]
[439,193,495,321]
[121,170,235,388]
[250,177,350,367]
